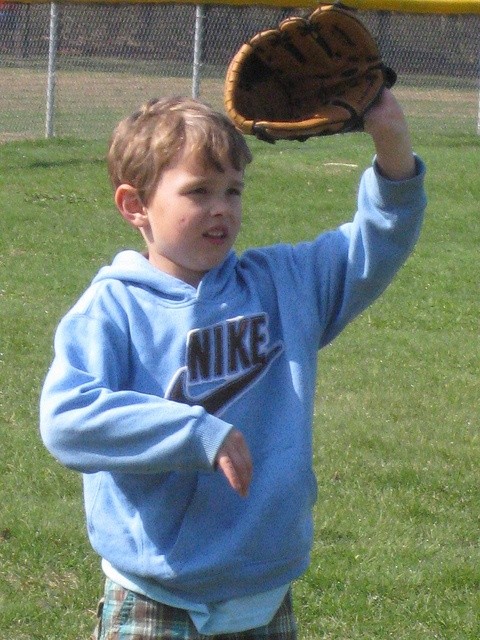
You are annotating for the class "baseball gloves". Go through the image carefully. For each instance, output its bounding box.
[224,5,398,146]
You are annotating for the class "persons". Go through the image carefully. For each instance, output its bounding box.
[38,5,426,640]
